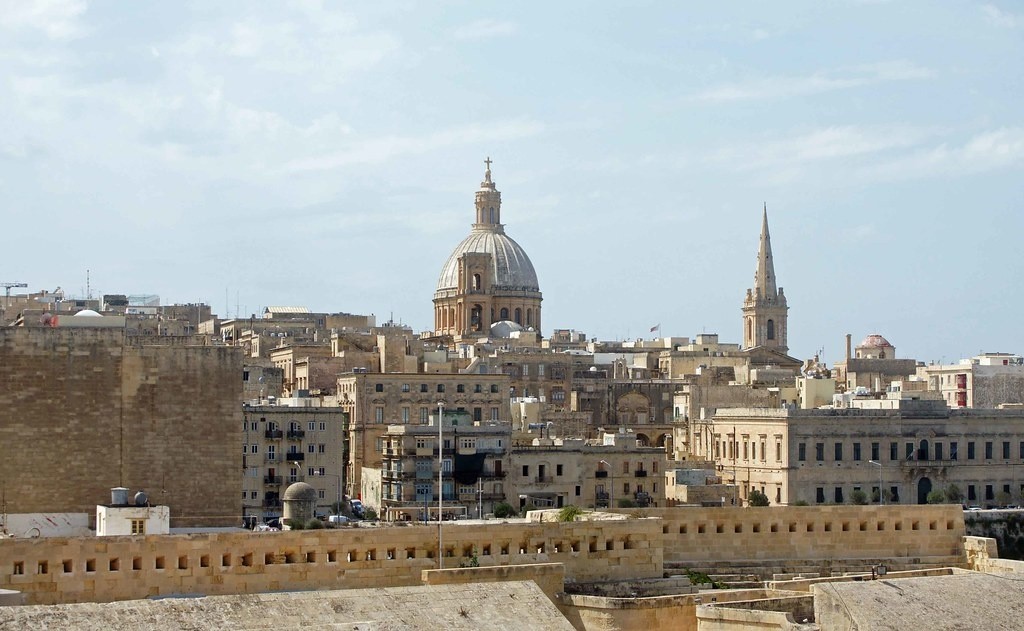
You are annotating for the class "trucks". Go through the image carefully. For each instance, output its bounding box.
[328,516,351,522]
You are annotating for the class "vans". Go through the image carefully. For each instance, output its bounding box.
[351,500,362,510]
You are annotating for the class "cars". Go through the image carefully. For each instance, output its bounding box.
[265,519,282,528]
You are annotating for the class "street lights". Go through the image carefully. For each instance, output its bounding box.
[869,460,883,504]
[434,397,448,568]
[600,459,614,509]
[293,461,301,481]
